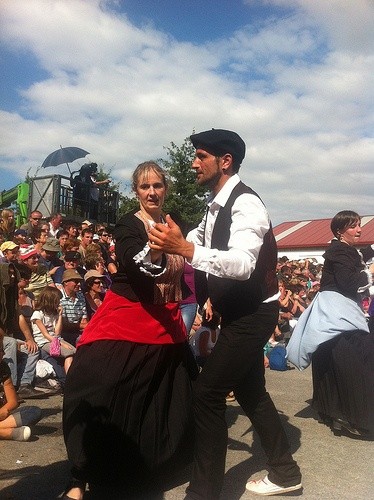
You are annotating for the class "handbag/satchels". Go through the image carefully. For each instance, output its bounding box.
[50,337,61,358]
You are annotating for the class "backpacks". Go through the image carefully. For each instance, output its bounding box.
[269,346,295,371]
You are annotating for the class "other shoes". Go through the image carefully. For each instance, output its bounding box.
[16,383,47,398]
[62,480,86,500]
[32,377,54,388]
[15,426,31,441]
[0,394,24,405]
[226,395,235,401]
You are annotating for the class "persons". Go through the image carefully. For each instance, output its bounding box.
[147,128,302,499]
[0,128,374,500]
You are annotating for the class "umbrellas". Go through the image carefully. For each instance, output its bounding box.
[41,144,90,173]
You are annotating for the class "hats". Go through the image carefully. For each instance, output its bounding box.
[190,129,246,163]
[19,244,37,259]
[1,241,19,251]
[63,251,81,260]
[60,269,84,284]
[15,229,28,236]
[83,269,107,282]
[42,238,62,252]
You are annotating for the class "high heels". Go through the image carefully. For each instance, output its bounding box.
[333,415,371,437]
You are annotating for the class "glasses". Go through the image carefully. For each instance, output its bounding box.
[28,254,41,259]
[71,280,83,284]
[101,233,111,237]
[95,280,105,285]
[33,218,41,220]
[66,258,77,262]
[12,247,20,254]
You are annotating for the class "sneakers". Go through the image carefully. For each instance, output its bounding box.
[246,474,301,495]
